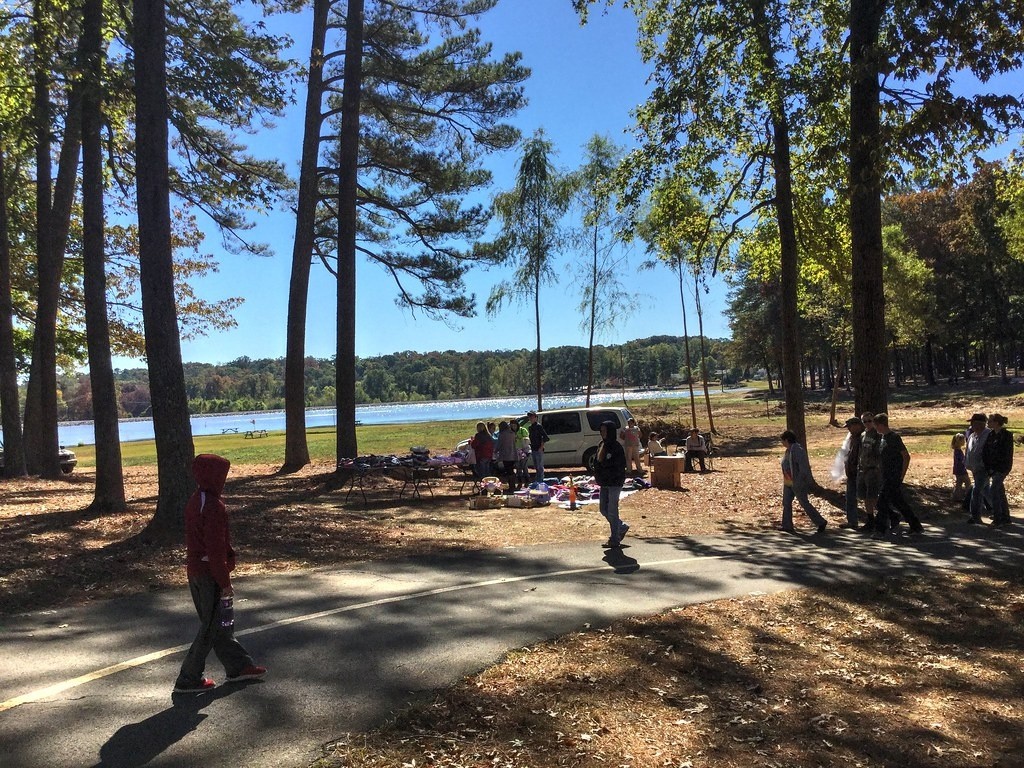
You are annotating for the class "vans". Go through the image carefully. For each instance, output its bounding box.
[455,406,640,473]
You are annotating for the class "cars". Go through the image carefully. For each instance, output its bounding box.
[0,423,77,475]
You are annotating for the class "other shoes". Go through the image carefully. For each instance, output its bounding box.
[601,537,620,548]
[839,521,925,540]
[620,522,629,542]
[778,526,794,531]
[816,520,828,533]
[967,517,983,524]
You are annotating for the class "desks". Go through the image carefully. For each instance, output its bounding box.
[453,454,533,495]
[339,465,445,503]
[221,427,238,434]
[355,421,363,427]
[244,429,267,440]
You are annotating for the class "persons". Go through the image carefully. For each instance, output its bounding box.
[494,421,520,496]
[527,412,550,483]
[777,431,827,532]
[950,433,971,512]
[648,432,667,456]
[839,413,924,539]
[621,418,646,476]
[685,429,708,474]
[593,421,629,547]
[963,413,1013,528]
[470,422,494,478]
[510,420,532,487]
[486,422,500,477]
[175,453,269,693]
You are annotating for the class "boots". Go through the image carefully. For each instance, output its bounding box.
[508,474,516,491]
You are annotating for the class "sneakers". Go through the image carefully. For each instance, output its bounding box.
[173,679,217,693]
[225,665,269,680]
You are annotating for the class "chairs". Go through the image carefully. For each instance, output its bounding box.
[649,433,714,472]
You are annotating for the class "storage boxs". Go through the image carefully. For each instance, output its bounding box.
[651,455,685,489]
[468,491,550,510]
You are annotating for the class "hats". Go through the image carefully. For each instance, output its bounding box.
[527,410,538,416]
[967,414,988,422]
[843,417,860,428]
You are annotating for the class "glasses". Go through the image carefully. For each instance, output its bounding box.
[863,419,872,423]
